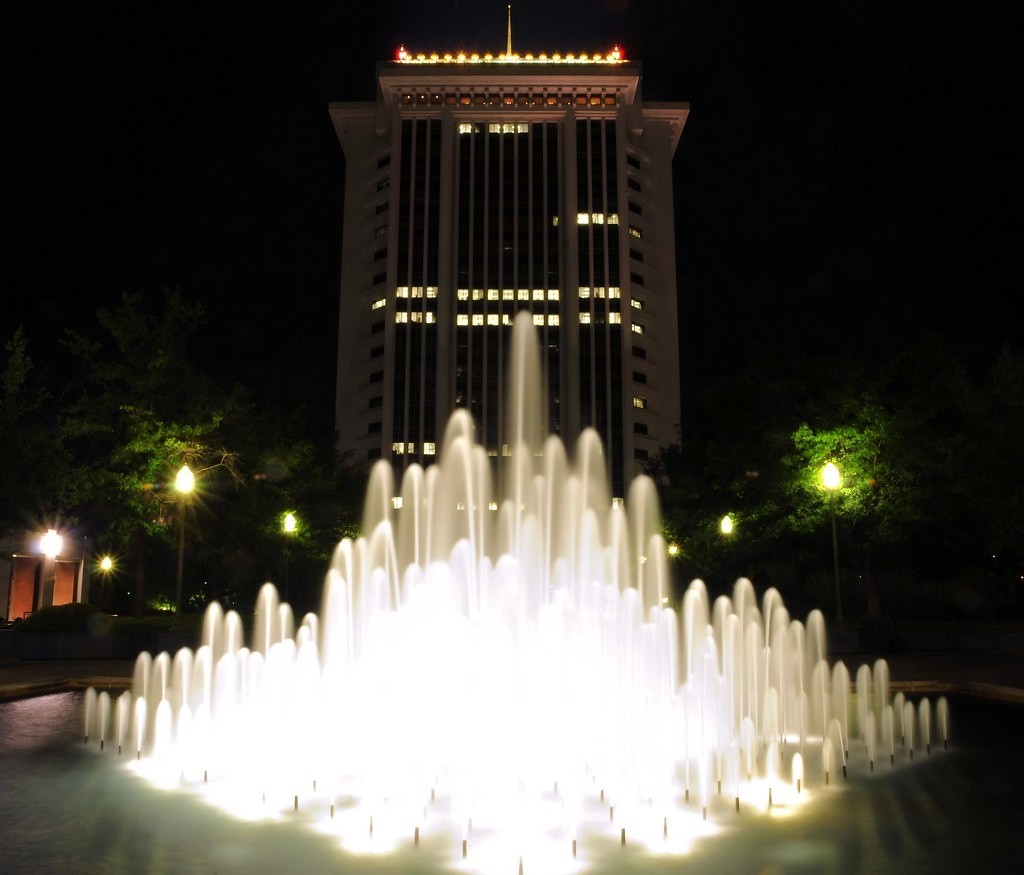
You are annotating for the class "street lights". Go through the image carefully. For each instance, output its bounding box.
[669,544,678,561]
[38,526,61,611]
[281,514,295,599]
[821,462,842,634]
[720,515,733,589]
[99,557,111,607]
[174,463,194,619]
